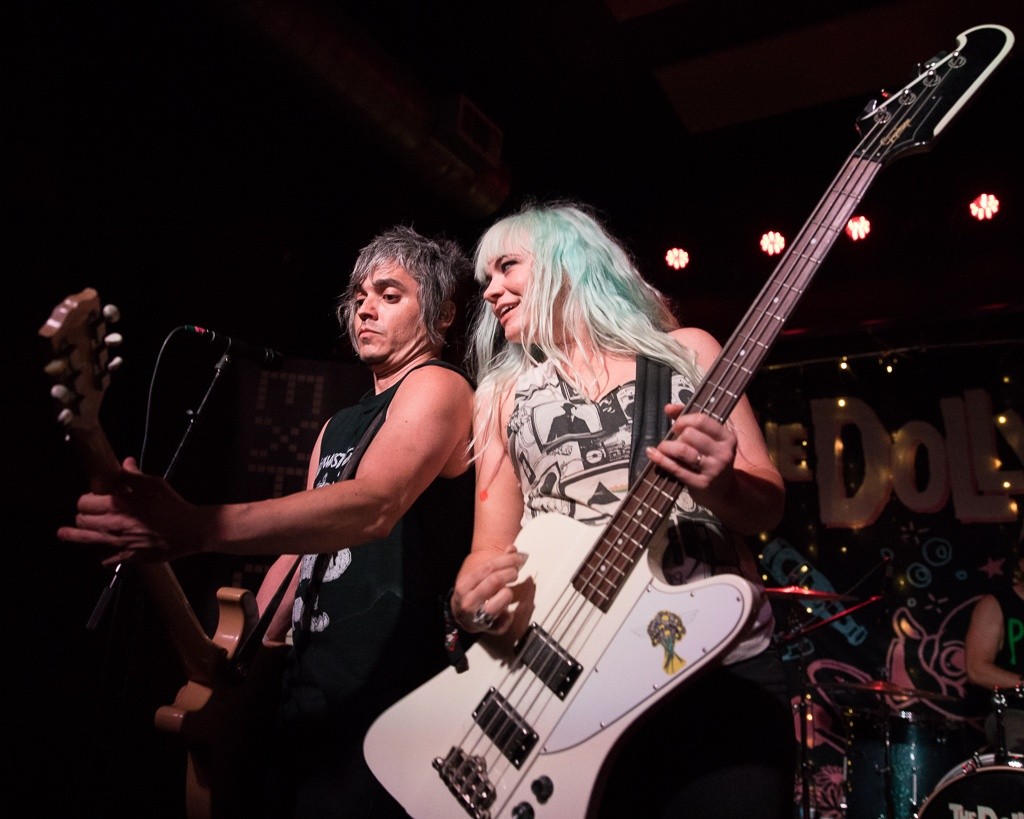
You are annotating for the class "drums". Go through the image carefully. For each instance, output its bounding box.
[915,750,1024,819]
[842,709,987,819]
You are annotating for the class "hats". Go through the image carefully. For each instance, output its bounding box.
[782,560,800,576]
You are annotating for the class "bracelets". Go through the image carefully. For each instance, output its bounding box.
[444,588,470,651]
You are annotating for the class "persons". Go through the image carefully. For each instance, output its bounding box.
[965,526,1024,754]
[58,224,479,819]
[450,202,786,819]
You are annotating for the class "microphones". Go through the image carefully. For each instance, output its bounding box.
[185,326,285,369]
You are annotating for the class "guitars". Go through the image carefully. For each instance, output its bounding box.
[38,285,288,731]
[361,21,1019,819]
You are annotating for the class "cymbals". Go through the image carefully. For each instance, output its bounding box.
[805,678,958,704]
[765,586,858,603]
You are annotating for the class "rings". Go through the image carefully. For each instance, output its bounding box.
[470,610,492,633]
[693,451,703,470]
